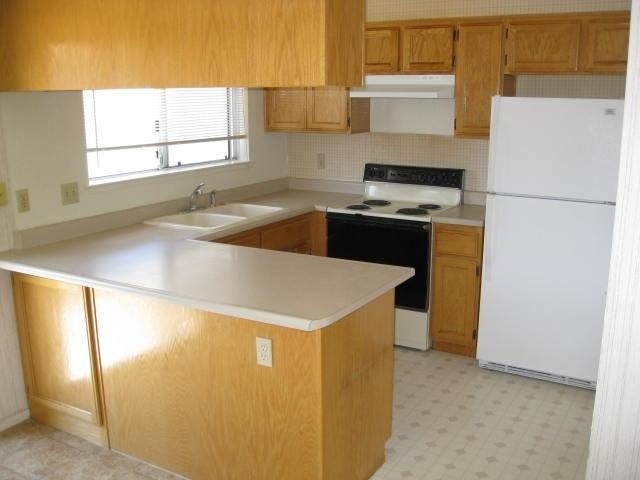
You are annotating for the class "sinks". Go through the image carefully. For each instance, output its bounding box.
[205,203,284,219]
[145,212,244,230]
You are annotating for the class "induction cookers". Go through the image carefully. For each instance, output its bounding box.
[326,197,457,222]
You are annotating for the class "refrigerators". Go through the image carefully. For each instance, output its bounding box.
[475,94,624,391]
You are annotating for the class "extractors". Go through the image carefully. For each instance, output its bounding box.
[349,74,455,99]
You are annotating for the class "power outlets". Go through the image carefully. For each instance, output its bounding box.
[255,336,272,368]
[61,182,80,205]
[317,153,325,171]
[0,183,10,210]
[16,188,31,214]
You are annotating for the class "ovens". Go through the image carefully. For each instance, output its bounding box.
[326,213,431,352]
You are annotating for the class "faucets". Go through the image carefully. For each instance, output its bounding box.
[189,183,205,211]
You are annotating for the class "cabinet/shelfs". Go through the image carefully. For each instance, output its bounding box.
[450,18,516,139]
[426,223,486,357]
[510,10,631,77]
[263,86,370,135]
[212,210,329,256]
[7,273,103,478]
[364,21,456,78]
